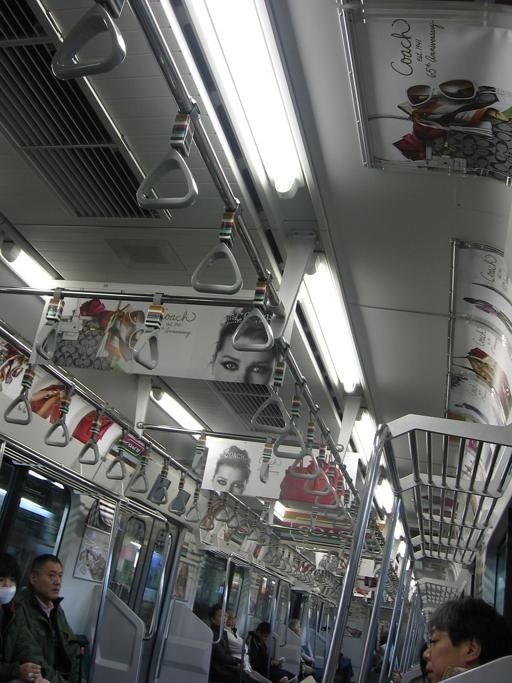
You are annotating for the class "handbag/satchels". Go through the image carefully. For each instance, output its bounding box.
[300,657,314,678]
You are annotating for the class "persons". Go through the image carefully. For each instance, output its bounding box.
[209,603,259,682]
[287,618,318,681]
[421,595,512,683]
[213,446,253,494]
[0,551,49,682]
[12,554,88,682]
[224,606,290,683]
[211,309,277,388]
[375,638,401,683]
[245,621,296,682]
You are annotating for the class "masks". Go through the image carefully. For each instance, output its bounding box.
[0,586,17,605]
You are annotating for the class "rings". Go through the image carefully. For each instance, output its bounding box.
[28,672,33,678]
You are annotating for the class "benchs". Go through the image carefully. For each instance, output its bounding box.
[160,600,329,682]
